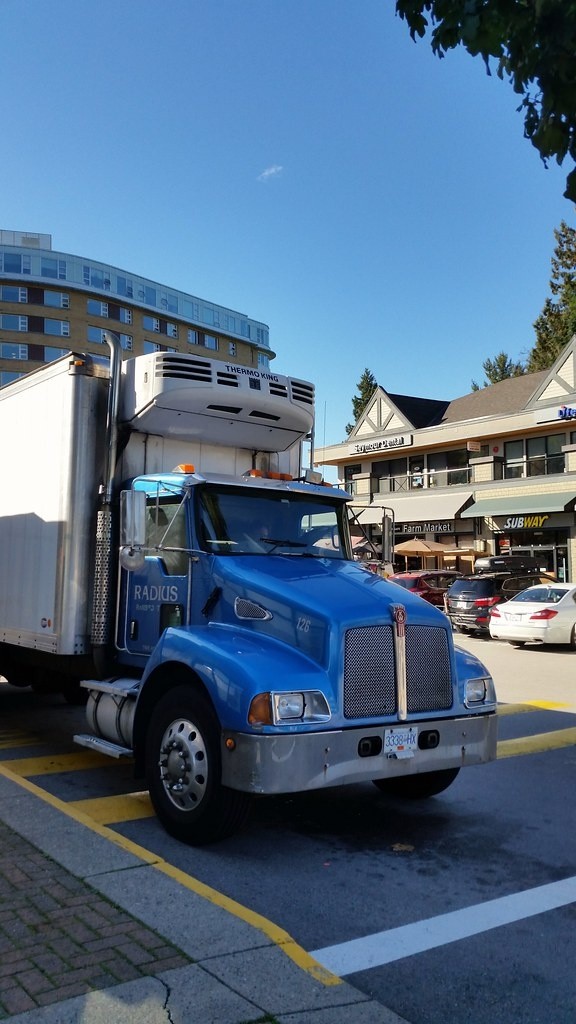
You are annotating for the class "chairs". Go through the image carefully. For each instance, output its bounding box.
[544,597,554,603]
[262,503,300,550]
[525,596,535,601]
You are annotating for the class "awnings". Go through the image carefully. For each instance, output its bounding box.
[354,493,472,524]
[460,490,576,518]
[298,508,364,529]
[312,530,366,551]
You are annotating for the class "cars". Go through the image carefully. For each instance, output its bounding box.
[386,568,466,611]
[489,583,576,651]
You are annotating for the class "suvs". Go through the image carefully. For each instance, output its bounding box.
[442,555,567,634]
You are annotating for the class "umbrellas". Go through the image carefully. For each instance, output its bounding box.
[389,536,460,557]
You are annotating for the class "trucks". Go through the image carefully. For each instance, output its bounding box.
[0,330,499,841]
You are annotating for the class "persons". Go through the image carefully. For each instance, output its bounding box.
[244,518,274,548]
